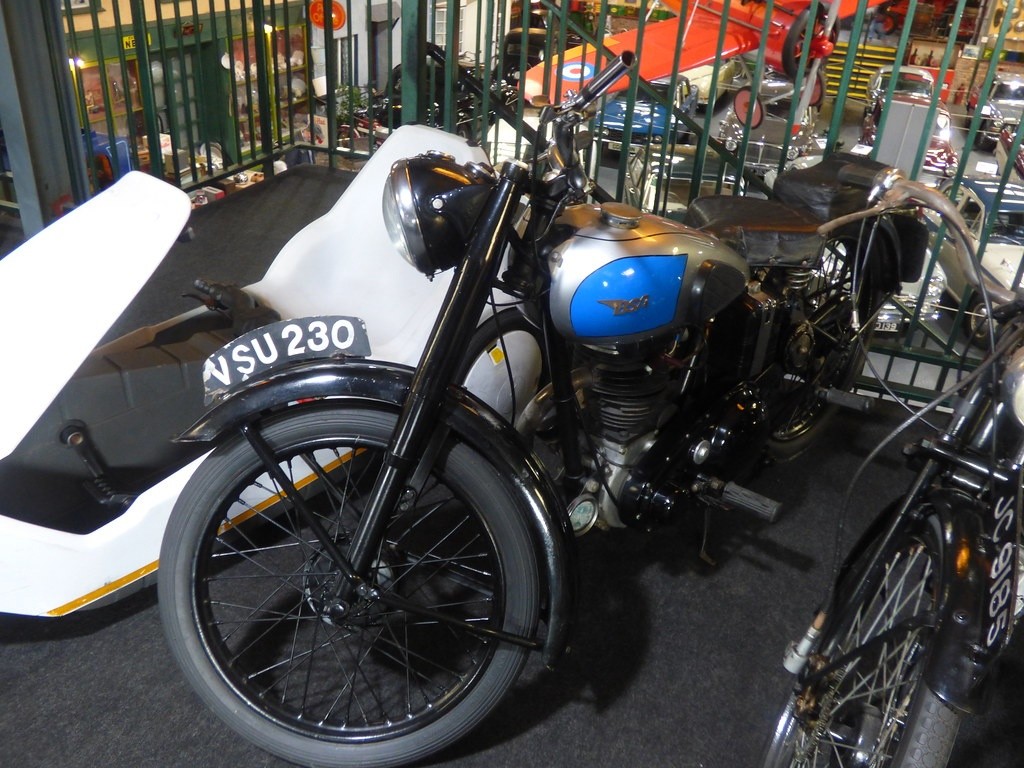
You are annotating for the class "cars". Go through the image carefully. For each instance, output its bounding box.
[650,57,734,106]
[364,57,524,148]
[868,64,941,104]
[587,73,699,158]
[994,118,1023,181]
[864,92,959,178]
[969,69,1023,148]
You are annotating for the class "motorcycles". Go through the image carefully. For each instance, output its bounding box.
[155,30,1024,767]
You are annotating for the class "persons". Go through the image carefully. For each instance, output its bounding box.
[283,121,325,169]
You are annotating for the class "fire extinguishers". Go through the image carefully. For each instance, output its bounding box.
[953,83,966,105]
